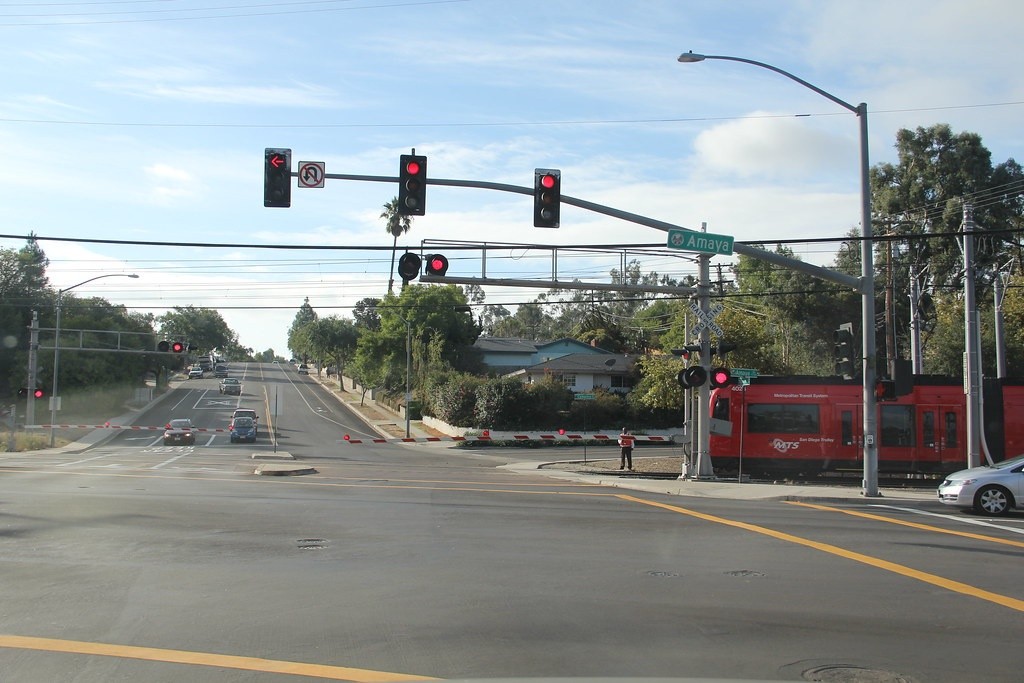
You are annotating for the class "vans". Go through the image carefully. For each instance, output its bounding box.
[230,408,259,427]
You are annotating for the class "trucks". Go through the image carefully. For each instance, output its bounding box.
[198,356,213,372]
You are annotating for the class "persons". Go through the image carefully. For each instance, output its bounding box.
[618,427,635,469]
[326,369,330,378]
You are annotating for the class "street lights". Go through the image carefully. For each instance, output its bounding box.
[678,50,877,497]
[49,274,139,448]
[369,307,411,440]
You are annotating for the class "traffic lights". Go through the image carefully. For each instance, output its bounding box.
[710,341,737,359]
[36,390,43,399]
[833,323,857,380]
[264,148,291,207]
[399,155,427,216]
[534,168,561,228]
[158,341,199,353]
[679,366,730,388]
[398,253,449,281]
[670,345,700,359]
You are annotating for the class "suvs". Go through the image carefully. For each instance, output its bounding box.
[164,418,199,445]
[297,364,308,374]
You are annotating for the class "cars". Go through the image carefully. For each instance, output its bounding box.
[219,378,241,396]
[272,360,296,366]
[936,454,1024,517]
[188,364,229,379]
[217,357,226,363]
[229,417,256,442]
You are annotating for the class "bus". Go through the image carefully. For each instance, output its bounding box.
[707,373,1024,482]
